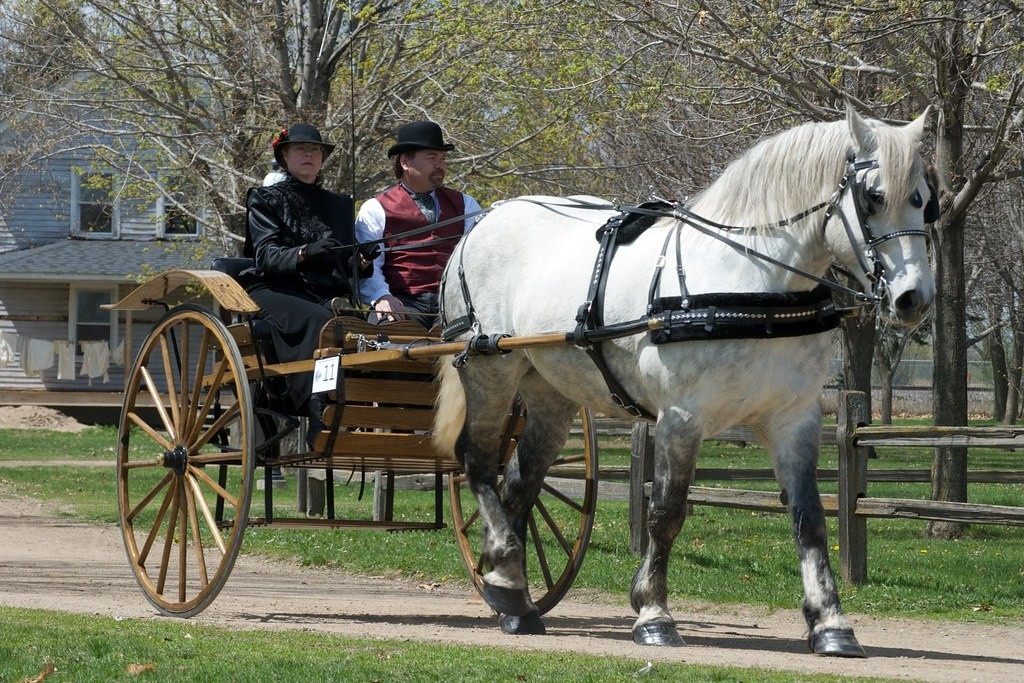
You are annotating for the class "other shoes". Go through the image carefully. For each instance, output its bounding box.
[304,398,330,452]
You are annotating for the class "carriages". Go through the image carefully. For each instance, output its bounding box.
[99,101,947,658]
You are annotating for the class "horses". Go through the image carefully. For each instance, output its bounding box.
[430,104,936,661]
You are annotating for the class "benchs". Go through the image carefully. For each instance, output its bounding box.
[213,257,308,373]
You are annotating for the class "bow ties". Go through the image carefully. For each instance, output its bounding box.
[410,192,436,224]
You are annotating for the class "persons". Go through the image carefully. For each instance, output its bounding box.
[237,123,380,442]
[351,120,486,433]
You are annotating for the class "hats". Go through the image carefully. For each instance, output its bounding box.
[272,123,335,170]
[387,121,455,159]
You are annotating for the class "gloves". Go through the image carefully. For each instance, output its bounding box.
[358,239,382,263]
[303,237,343,263]
